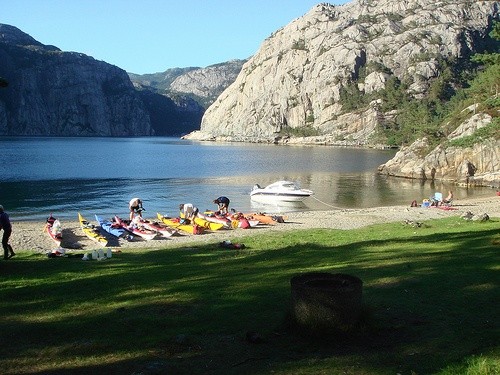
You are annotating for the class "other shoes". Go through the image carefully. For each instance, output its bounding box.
[2,253,15,260]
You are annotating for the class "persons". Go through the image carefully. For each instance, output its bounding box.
[214,196,230,214]
[440,190,453,207]
[129,198,146,219]
[179,203,199,225]
[0,205,16,259]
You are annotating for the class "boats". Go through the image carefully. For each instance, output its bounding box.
[45,213,64,245]
[114,213,158,241]
[78,211,108,246]
[206,210,259,229]
[95,215,134,242]
[157,210,204,236]
[231,208,289,225]
[132,212,176,240]
[248,178,316,203]
[181,211,223,235]
